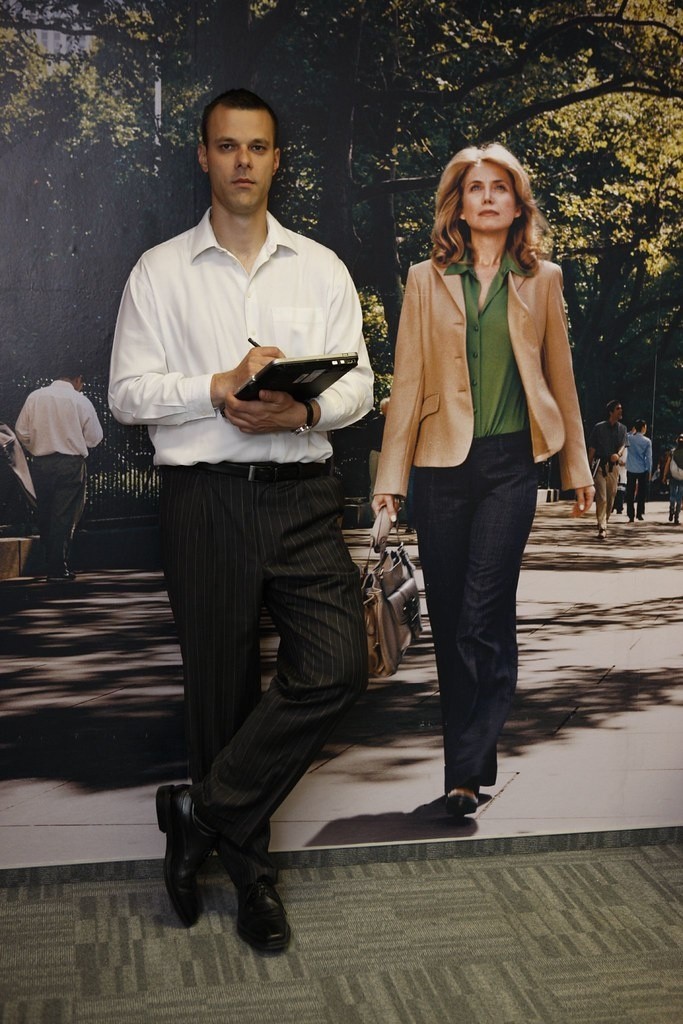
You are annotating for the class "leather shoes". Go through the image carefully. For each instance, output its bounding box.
[231,874,292,951]
[156,783,215,928]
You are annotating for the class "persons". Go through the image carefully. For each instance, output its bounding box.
[371,146,594,826]
[13,363,683,585]
[108,88,373,956]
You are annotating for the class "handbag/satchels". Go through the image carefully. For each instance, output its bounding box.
[359,499,422,678]
[671,458,683,481]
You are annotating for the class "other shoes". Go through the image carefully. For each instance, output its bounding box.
[669,512,673,521]
[636,514,643,520]
[47,567,76,581]
[630,516,634,522]
[445,788,479,818]
[598,529,607,538]
[675,521,681,526]
[617,509,622,514]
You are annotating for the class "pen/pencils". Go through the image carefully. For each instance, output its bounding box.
[247,337,262,347]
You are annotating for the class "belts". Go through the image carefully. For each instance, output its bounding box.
[190,462,330,483]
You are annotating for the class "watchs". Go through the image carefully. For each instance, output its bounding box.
[292,402,314,437]
